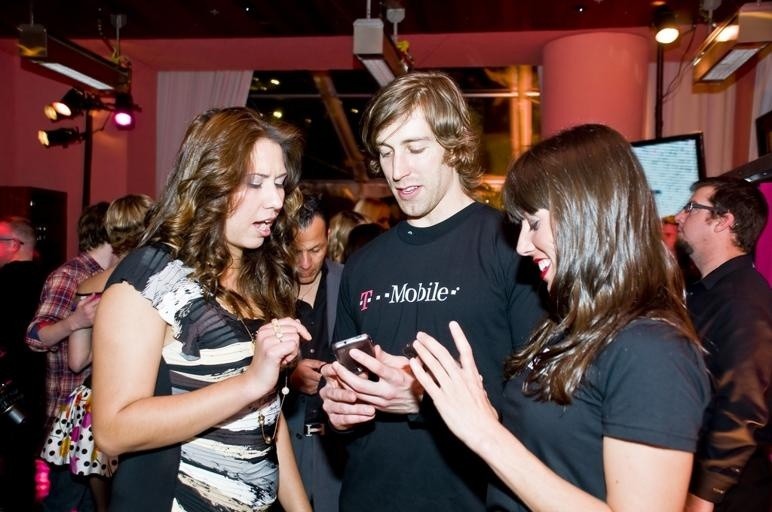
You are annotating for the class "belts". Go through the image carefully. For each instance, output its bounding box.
[304,424,330,435]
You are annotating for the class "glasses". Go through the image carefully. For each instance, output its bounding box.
[685,202,715,213]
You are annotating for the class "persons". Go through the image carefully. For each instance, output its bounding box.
[92,105,317,511]
[660,175,772,511]
[313,71,560,512]
[407,121,713,512]
[2,180,402,510]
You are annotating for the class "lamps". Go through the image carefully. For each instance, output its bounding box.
[652,6,696,47]
[352,0,408,90]
[690,0,772,94]
[18,0,130,92]
[36,87,143,148]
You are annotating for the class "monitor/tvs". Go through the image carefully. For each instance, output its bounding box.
[630,131,706,220]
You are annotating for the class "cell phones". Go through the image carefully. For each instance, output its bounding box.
[332,333,376,376]
